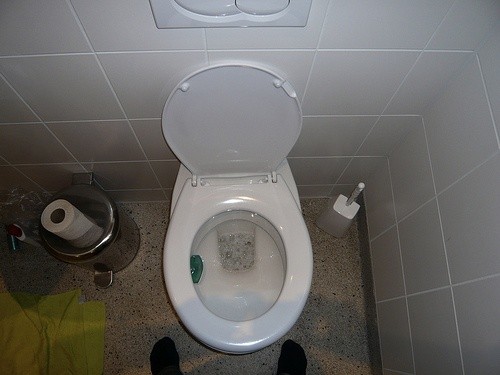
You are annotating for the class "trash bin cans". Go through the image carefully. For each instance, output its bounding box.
[37,172,141,289]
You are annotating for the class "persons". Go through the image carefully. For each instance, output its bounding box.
[149,337,308,375]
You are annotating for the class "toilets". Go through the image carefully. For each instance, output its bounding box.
[161,59,314,355]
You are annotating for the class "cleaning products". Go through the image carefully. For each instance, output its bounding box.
[6,224,41,248]
